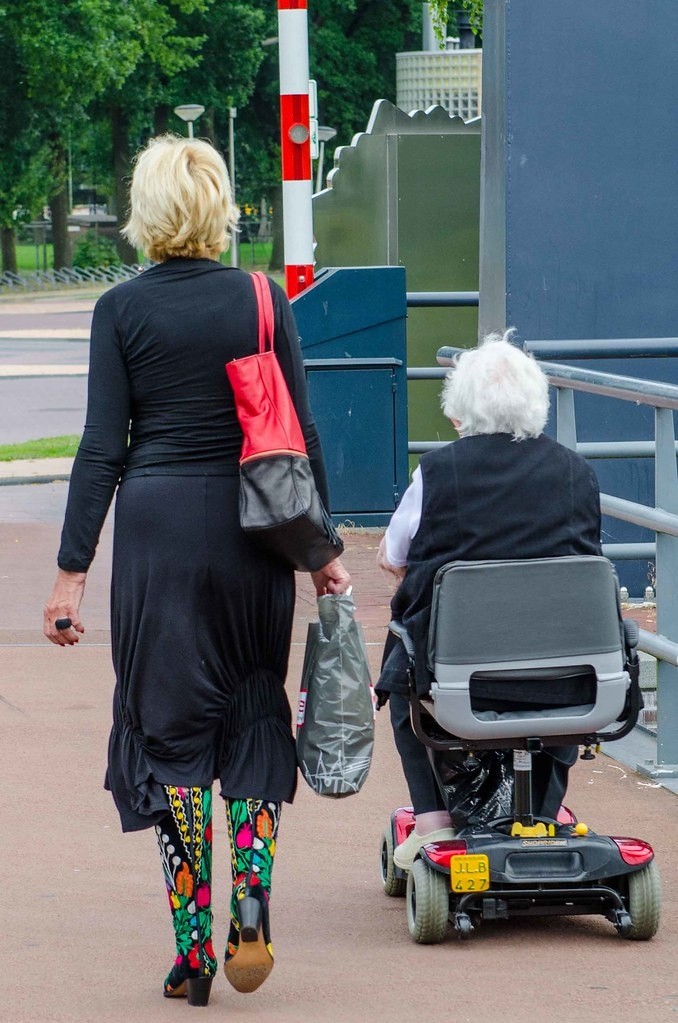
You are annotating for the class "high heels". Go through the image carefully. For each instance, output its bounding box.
[163,955,217,1006]
[223,885,275,993]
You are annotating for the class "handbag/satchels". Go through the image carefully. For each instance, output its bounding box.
[295,595,378,799]
[224,272,345,573]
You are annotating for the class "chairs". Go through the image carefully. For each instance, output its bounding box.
[388,555,644,760]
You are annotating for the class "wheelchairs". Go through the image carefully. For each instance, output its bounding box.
[377,555,661,946]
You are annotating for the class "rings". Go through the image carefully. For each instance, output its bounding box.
[55,618,73,629]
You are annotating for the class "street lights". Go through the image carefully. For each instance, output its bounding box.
[313,126,338,198]
[174,104,209,140]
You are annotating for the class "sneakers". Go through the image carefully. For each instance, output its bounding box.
[393,828,455,869]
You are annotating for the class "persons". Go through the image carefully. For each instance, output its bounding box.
[372,326,644,873]
[44,133,352,1007]
